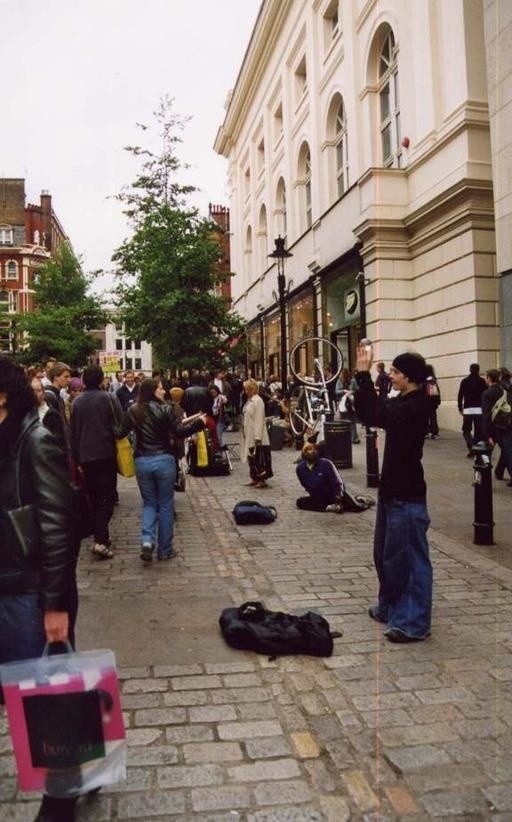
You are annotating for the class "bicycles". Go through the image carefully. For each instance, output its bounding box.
[283,333,345,439]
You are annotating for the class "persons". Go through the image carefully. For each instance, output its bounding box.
[458,363,512,487]
[0,357,92,820]
[1,355,442,561]
[347,340,436,647]
[112,374,212,563]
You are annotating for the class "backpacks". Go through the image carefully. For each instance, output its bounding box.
[491,389,512,429]
[234,500,278,526]
[220,485,343,660]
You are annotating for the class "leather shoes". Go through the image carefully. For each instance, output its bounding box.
[35,794,81,822]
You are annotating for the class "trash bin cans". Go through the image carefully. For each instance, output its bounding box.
[323,420,353,469]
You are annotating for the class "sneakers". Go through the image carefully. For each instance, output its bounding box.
[356,494,376,506]
[157,548,176,561]
[329,503,344,513]
[140,543,152,562]
[94,543,114,558]
[386,630,431,644]
[368,606,388,622]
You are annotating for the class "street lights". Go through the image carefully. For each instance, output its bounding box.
[267,239,297,404]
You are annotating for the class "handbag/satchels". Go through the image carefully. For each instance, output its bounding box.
[115,436,135,478]
[1,639,128,797]
[247,441,273,480]
[196,430,209,467]
[8,486,99,560]
